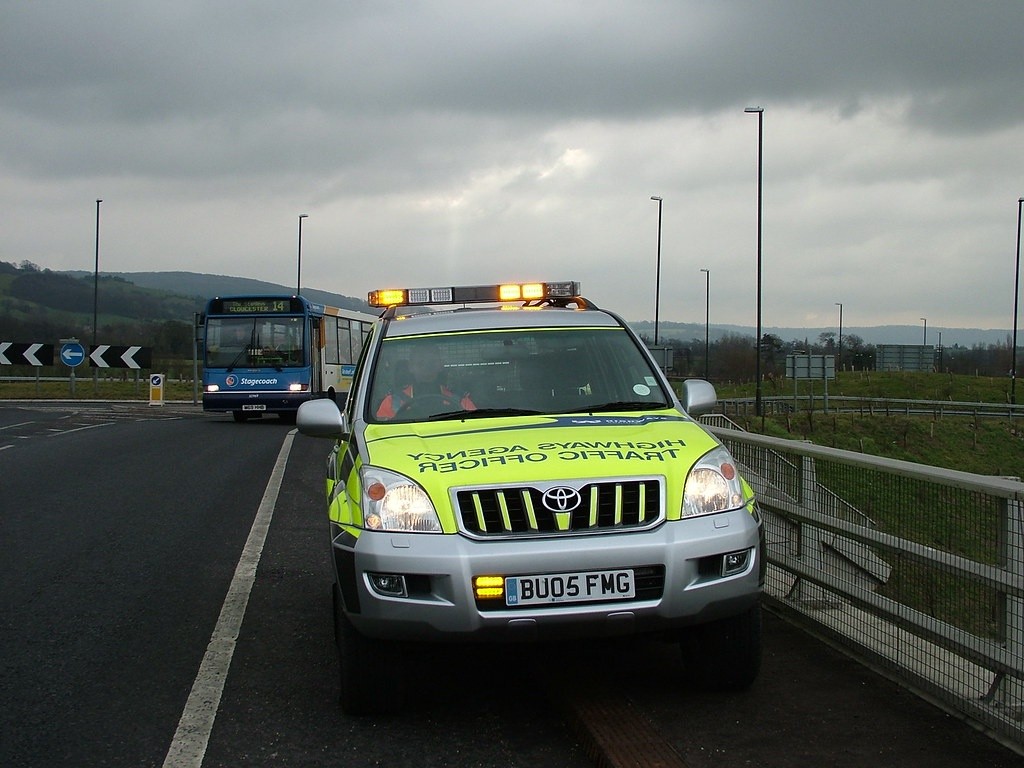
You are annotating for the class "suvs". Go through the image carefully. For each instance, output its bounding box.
[295,280,767,698]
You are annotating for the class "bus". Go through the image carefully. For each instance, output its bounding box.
[199,292,383,423]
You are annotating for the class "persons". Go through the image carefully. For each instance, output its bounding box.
[216,324,361,365]
[375,341,479,420]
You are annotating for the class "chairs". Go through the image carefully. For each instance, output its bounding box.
[528,355,594,412]
[392,358,449,391]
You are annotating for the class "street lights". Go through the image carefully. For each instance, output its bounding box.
[89,208,105,385]
[297,213,309,293]
[744,107,766,416]
[836,303,843,372]
[700,268,710,381]
[650,195,663,344]
[1008,197,1024,409]
[920,317,927,346]
